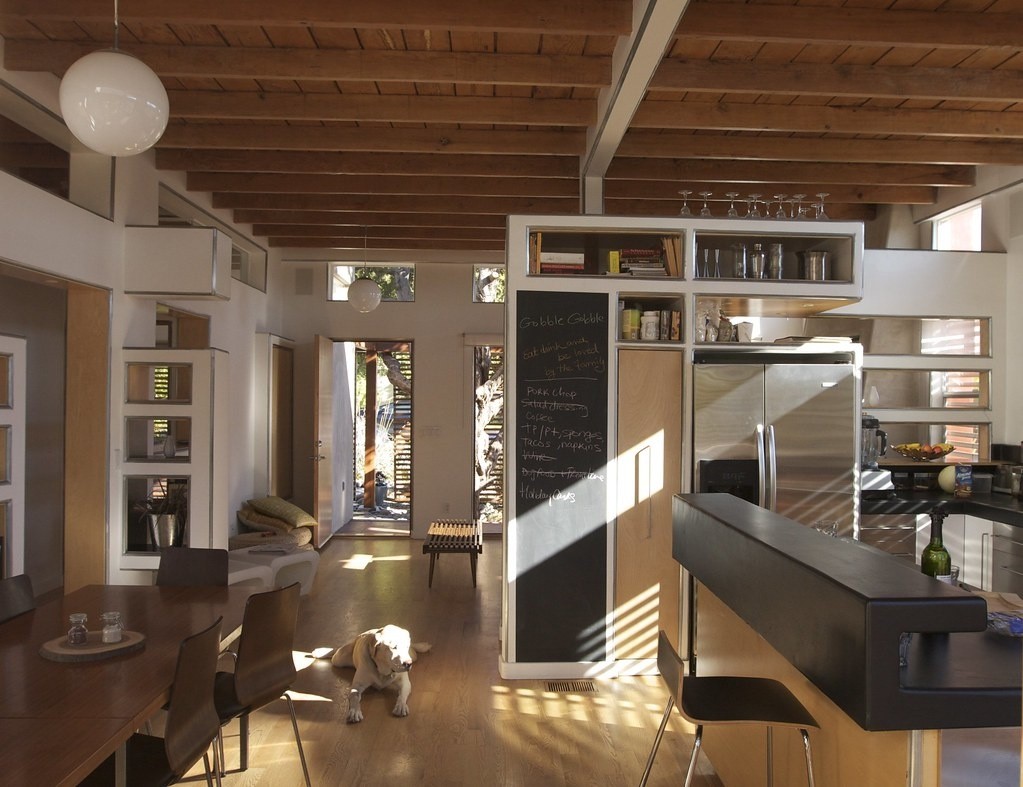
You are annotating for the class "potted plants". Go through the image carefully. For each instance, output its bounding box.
[130,478,187,551]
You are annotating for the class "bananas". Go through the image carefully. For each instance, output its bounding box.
[934,443,953,452]
[896,443,920,451]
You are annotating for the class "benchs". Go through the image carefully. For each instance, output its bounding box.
[423,519,482,588]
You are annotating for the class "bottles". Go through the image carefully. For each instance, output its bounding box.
[163,435,176,459]
[101,611,124,643]
[749,243,766,277]
[640,311,660,340]
[921,521,951,581]
[67,613,89,646]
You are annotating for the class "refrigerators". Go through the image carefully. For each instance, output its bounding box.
[692,358,859,539]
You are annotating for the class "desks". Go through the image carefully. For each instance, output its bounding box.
[0,585,282,787]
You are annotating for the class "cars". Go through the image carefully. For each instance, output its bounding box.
[358,401,412,437]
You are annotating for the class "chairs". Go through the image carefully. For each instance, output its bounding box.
[156,547,229,587]
[146,581,312,787]
[0,574,36,625]
[76,616,224,787]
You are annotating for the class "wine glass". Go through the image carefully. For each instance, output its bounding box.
[698,190,713,217]
[678,190,693,216]
[723,191,831,220]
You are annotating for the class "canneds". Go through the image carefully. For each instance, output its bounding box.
[622,308,641,339]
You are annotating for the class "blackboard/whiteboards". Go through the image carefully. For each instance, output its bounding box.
[514,289,609,662]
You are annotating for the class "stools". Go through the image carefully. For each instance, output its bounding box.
[229,542,320,595]
[639,630,821,787]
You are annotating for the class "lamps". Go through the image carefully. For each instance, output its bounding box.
[58,0,169,157]
[348,225,382,313]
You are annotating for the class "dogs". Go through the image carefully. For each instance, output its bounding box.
[311,624,432,724]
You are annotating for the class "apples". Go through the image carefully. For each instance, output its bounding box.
[920,444,942,453]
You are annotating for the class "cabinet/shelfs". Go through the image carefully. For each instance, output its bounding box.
[502,214,865,680]
[812,247,1007,464]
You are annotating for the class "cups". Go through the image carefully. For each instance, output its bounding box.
[815,519,838,538]
[862,418,888,470]
[768,243,784,279]
[731,243,749,278]
[803,250,832,280]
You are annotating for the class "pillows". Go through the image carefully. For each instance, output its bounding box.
[237,508,293,532]
[247,495,318,528]
